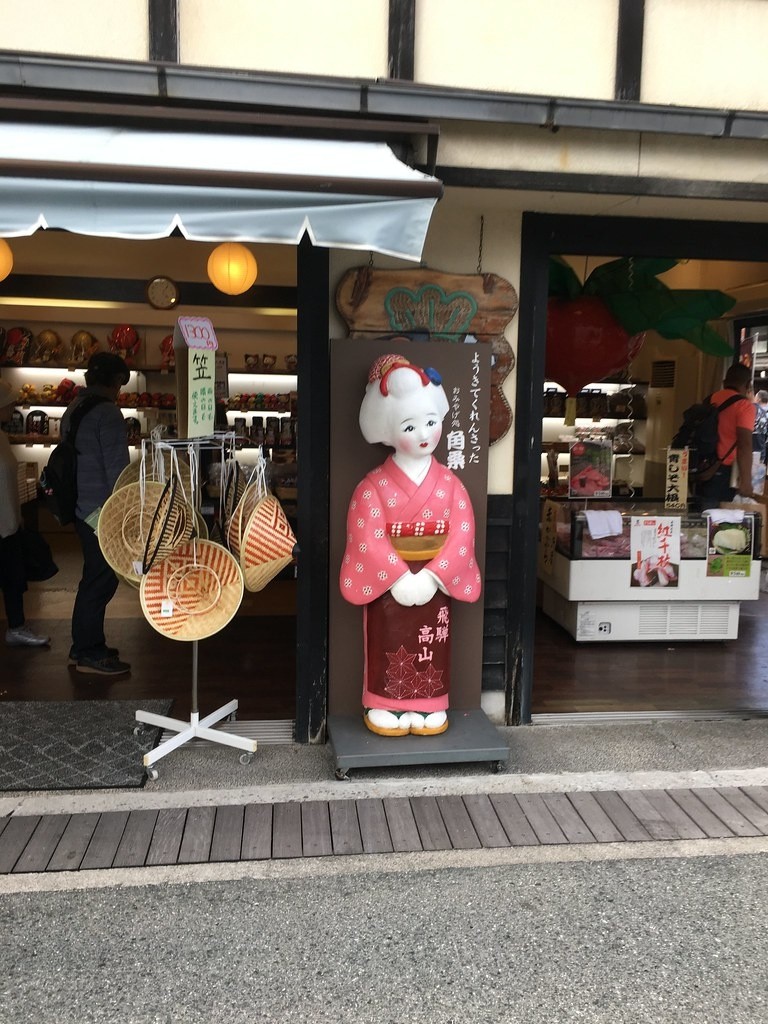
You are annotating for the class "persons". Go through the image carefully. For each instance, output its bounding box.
[693,361,755,512]
[751,389,768,476]
[0,380,51,649]
[54,350,134,676]
[339,354,483,736]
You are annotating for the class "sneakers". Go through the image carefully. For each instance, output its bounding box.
[67,640,120,664]
[76,656,131,676]
[4,625,51,645]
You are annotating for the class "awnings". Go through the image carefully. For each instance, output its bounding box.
[0,97,442,264]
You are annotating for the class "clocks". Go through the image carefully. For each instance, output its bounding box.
[145,275,179,309]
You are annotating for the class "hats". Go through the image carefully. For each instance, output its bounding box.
[97,452,209,592]
[8,528,59,581]
[0,378,21,408]
[224,461,301,593]
[139,538,244,642]
[87,352,130,384]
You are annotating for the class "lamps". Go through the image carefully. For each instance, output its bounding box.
[0,238,14,282]
[207,243,258,296]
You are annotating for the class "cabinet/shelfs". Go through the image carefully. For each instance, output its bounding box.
[0,324,177,515]
[539,388,648,542]
[227,354,299,503]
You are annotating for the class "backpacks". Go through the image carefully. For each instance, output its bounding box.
[36,394,116,527]
[670,392,748,484]
